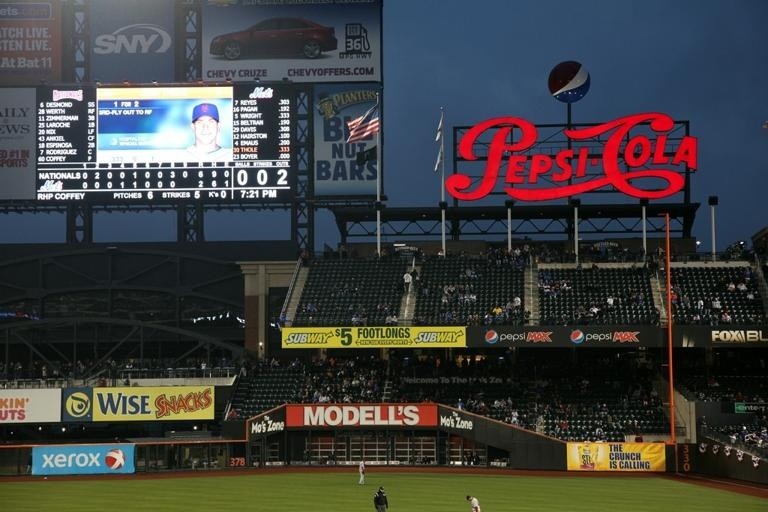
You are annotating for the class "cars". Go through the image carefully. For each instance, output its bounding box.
[210,17,339,62]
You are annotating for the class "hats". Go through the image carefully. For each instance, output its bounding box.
[380,486,384,489]
[192,103,220,122]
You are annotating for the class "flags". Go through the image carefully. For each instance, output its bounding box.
[345,104,380,144]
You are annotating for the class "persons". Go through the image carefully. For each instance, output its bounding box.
[358,458,367,485]
[373,487,388,512]
[466,495,479,512]
[228,408,236,419]
[255,239,768,468]
[177,103,232,161]
[0,349,254,398]
